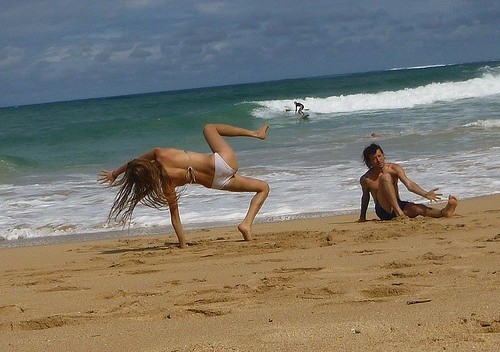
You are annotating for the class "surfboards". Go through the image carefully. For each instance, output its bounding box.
[302,114,309,118]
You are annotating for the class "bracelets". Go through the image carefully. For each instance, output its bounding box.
[111,170,116,179]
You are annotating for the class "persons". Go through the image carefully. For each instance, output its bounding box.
[294,101,305,116]
[357,143,457,221]
[97,124,270,249]
